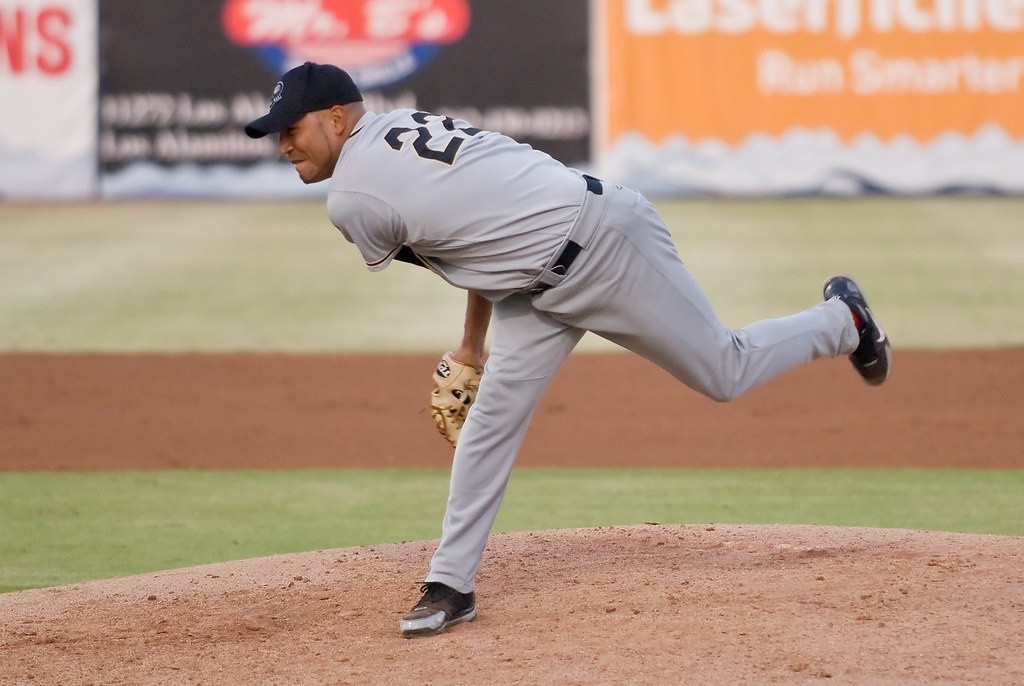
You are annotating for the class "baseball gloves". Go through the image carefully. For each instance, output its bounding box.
[427,351,486,449]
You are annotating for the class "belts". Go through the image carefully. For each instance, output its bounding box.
[530,175,603,297]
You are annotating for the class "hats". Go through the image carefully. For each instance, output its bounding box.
[245,61,363,139]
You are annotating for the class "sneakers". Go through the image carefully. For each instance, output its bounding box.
[823,274,891,386]
[399,581,475,637]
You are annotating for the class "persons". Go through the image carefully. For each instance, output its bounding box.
[244,61,892,636]
